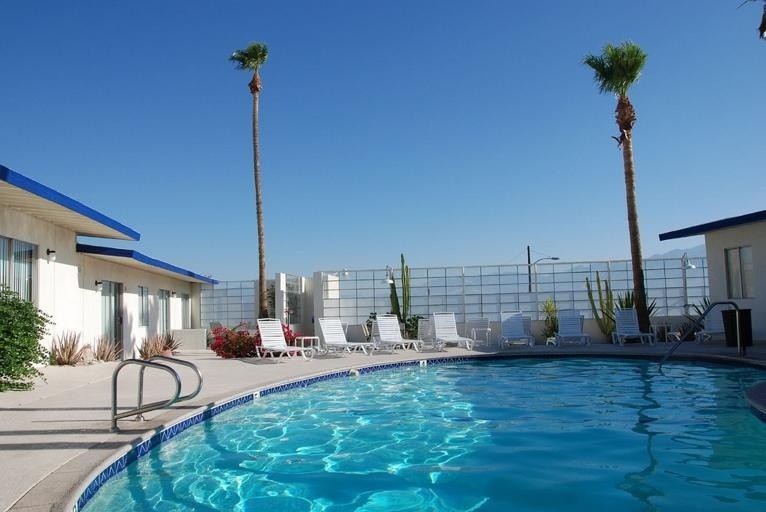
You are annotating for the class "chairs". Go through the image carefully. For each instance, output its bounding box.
[257,317,315,364]
[317,317,373,357]
[360,308,658,352]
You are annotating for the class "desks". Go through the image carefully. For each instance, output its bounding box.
[293,335,323,359]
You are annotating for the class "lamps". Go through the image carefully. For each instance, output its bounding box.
[46,248,56,261]
[95,280,103,291]
[171,290,177,299]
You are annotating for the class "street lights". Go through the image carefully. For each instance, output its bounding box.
[528,245,560,292]
[681,252,696,315]
[336,263,349,277]
[381,265,394,287]
